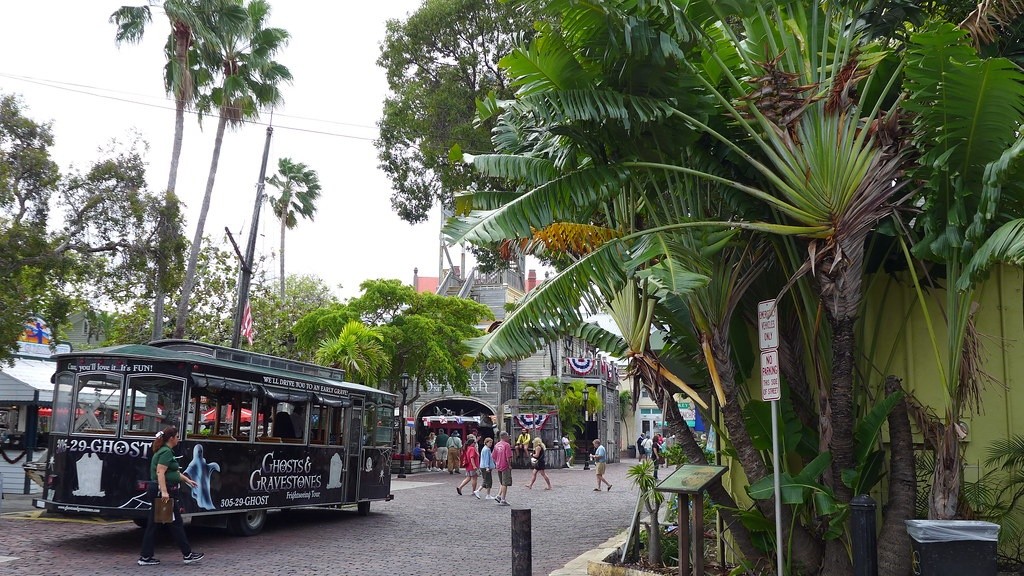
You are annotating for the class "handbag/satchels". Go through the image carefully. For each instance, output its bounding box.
[656,455,665,464]
[531,458,539,467]
[460,453,468,468]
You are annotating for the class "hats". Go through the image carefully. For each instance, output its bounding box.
[451,430,460,436]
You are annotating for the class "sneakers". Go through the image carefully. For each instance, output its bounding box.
[183,552,205,563]
[138,556,161,566]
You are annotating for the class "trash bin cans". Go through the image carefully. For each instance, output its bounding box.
[902,519,1001,576]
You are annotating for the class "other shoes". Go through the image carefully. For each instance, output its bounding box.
[566,462,571,467]
[608,485,612,491]
[639,462,642,464]
[525,484,532,489]
[474,491,481,499]
[500,500,511,506]
[486,495,494,499]
[426,467,446,472]
[495,496,501,502]
[593,489,601,491]
[450,471,453,474]
[455,470,461,474]
[457,487,462,495]
[655,478,661,481]
[547,483,551,490]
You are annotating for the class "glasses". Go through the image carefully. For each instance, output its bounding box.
[175,436,179,438]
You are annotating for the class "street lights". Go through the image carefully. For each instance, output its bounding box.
[581,388,590,470]
[397,371,410,478]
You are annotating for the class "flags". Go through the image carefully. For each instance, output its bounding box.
[407,417,462,427]
[240,297,252,345]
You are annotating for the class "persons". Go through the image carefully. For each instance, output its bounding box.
[456,438,479,495]
[467,428,481,451]
[414,428,449,474]
[515,428,530,458]
[473,437,495,500]
[562,432,574,468]
[290,406,313,439]
[589,439,612,491]
[525,437,551,490]
[651,436,661,481]
[637,434,676,467]
[446,430,462,475]
[491,431,513,505]
[137,428,205,565]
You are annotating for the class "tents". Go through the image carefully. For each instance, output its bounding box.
[114,411,144,420]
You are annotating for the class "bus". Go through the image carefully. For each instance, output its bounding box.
[32,337,399,537]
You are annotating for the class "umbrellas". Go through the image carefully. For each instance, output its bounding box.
[38,407,99,417]
[201,404,263,436]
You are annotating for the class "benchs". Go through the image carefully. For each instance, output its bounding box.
[85,428,323,444]
